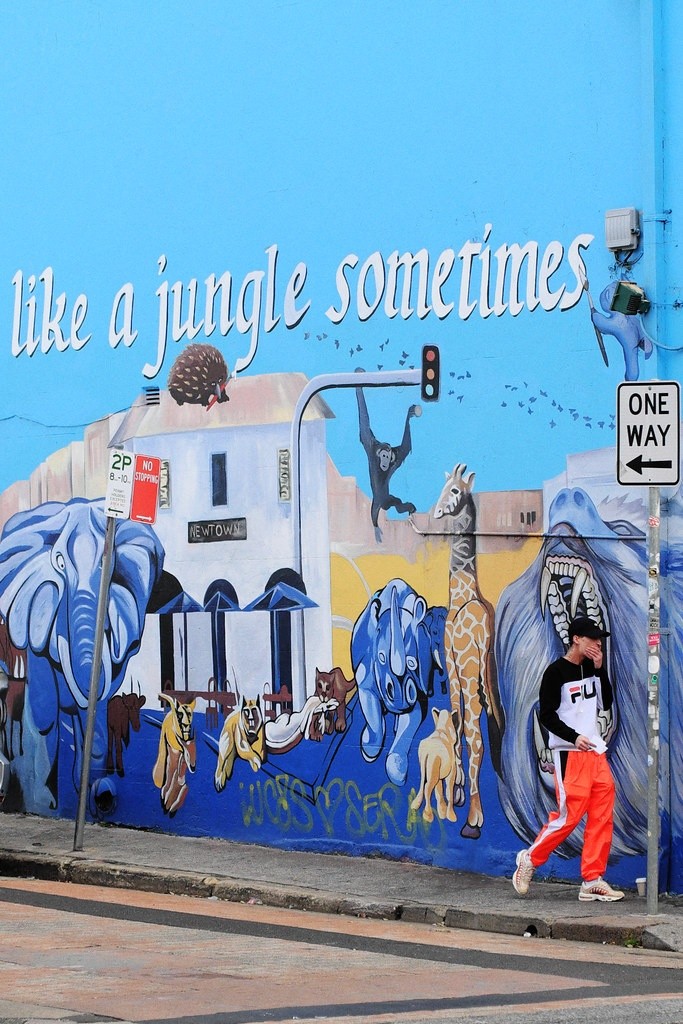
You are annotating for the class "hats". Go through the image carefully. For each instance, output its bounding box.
[567,617,612,639]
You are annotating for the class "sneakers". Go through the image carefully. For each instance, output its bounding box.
[512,849,537,896]
[577,876,625,902]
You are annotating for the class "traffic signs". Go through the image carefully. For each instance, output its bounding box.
[102,449,135,519]
[615,380,682,487]
[130,454,161,525]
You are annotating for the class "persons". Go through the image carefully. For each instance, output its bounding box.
[512,617,625,902]
